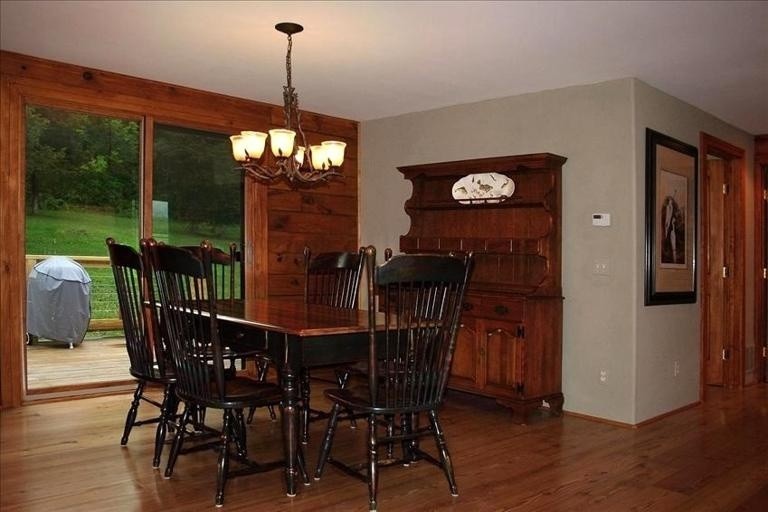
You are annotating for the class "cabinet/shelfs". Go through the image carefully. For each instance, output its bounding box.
[440,291,566,428]
[395,152,568,301]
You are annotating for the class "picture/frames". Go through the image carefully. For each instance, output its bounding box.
[644,124,699,308]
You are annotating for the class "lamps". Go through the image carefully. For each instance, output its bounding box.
[229,22,346,188]
